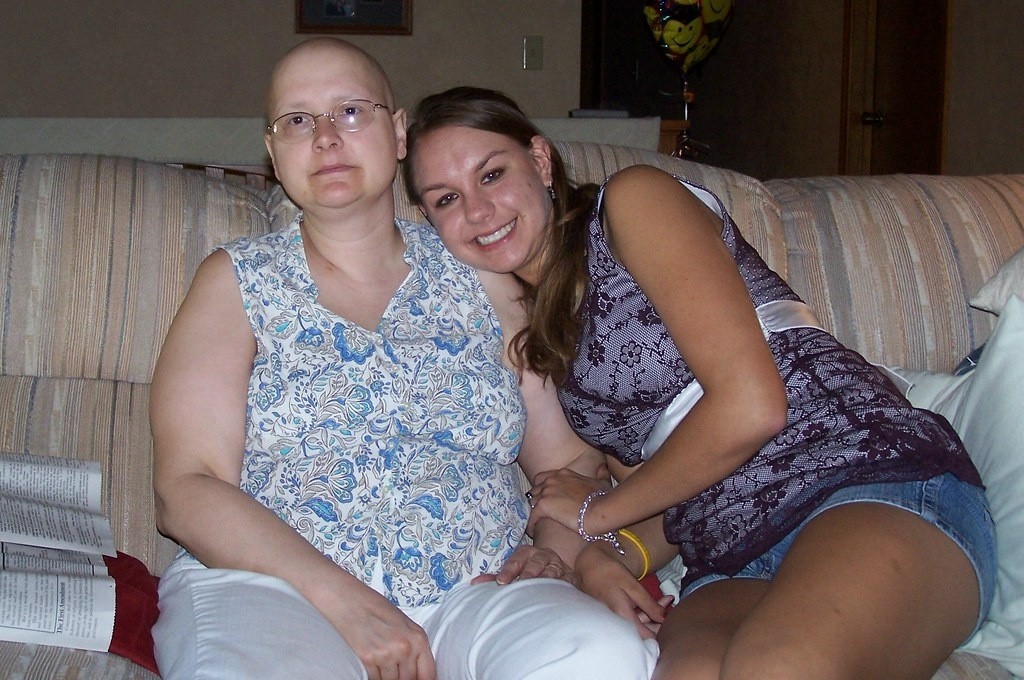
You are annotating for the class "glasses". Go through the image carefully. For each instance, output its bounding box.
[267,99,395,144]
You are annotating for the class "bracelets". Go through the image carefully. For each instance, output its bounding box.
[619,528,651,581]
[577,489,627,555]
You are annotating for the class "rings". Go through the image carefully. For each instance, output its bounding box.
[545,563,563,578]
[528,494,535,508]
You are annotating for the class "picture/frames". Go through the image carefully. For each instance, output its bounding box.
[294,0,413,36]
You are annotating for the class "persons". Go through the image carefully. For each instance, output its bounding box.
[400,85,1001,680]
[149,37,649,680]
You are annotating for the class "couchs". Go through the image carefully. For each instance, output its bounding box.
[0,142,1024,680]
